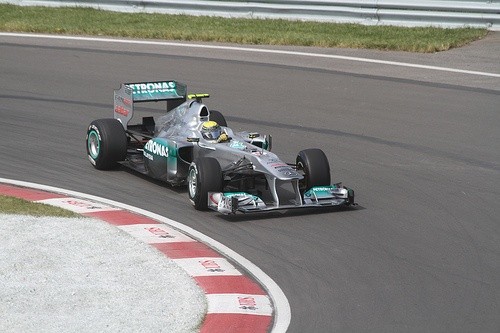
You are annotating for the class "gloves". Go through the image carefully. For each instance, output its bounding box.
[217,133,227,142]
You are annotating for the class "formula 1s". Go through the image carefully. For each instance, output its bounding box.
[86,80,358,216]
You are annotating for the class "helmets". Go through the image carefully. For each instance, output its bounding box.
[201,121,221,139]
